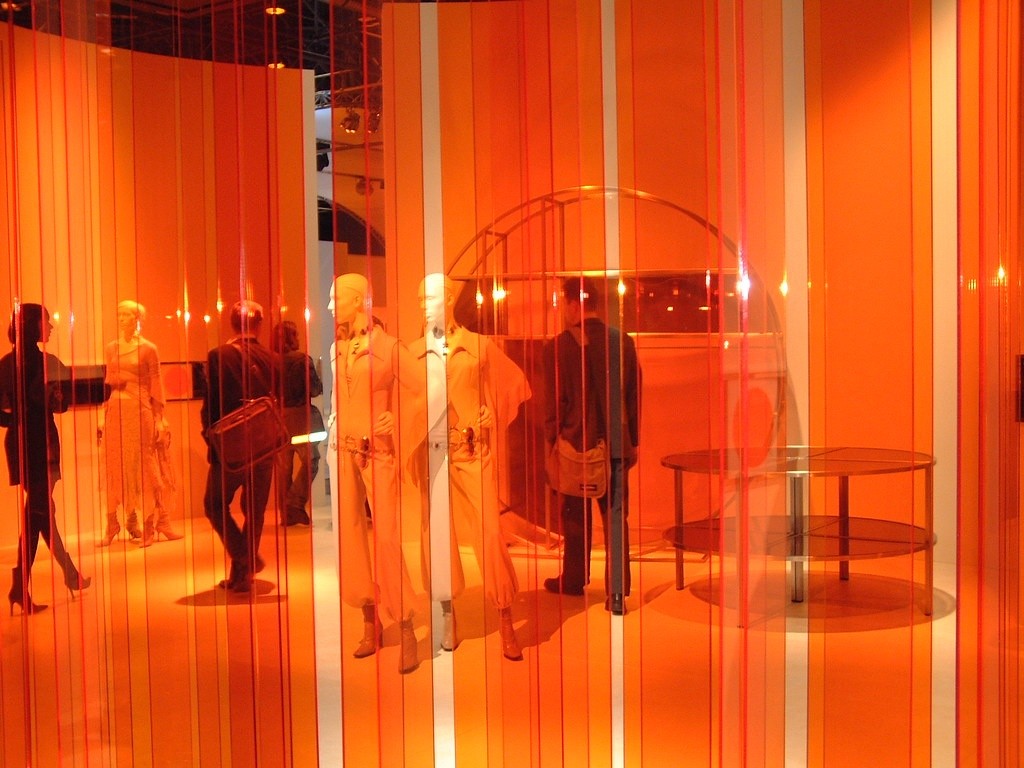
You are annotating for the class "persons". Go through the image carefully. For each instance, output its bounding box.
[204,300,280,592]
[96,300,183,547]
[543,276,641,613]
[266,320,325,528]
[0,303,127,617]
[325,273,533,672]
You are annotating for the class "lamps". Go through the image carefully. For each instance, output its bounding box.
[265,0,289,15]
[355,178,374,197]
[267,53,286,69]
[337,110,381,134]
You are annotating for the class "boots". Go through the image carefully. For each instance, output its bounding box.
[126,510,142,539]
[8,568,49,616]
[441,600,458,652]
[353,604,383,657]
[57,553,91,598]
[497,607,525,661]
[139,514,157,549]
[97,511,120,547]
[399,620,418,672]
[156,510,184,543]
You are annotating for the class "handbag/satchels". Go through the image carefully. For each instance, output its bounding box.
[206,397,291,473]
[548,431,612,500]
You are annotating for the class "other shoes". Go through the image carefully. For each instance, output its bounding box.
[286,503,309,526]
[280,514,296,527]
[250,556,265,575]
[220,579,249,593]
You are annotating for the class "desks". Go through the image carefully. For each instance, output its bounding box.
[661,445,938,617]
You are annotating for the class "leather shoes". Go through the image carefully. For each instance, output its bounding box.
[544,575,585,596]
[605,593,626,614]
